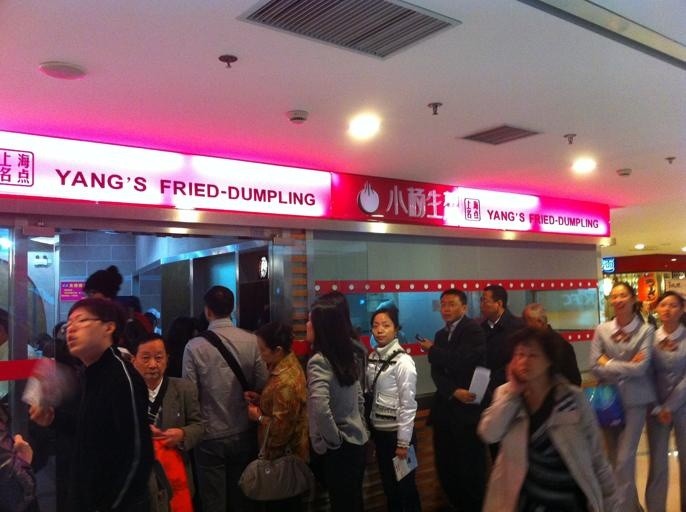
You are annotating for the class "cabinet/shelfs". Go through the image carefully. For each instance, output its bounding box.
[234,241,272,335]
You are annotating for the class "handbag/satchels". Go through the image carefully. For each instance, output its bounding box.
[581,381,626,431]
[237,451,319,503]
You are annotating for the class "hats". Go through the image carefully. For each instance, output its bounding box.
[82,264,123,301]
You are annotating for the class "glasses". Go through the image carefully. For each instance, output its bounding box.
[60,317,105,332]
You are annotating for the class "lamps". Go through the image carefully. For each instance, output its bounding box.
[36,61,86,79]
